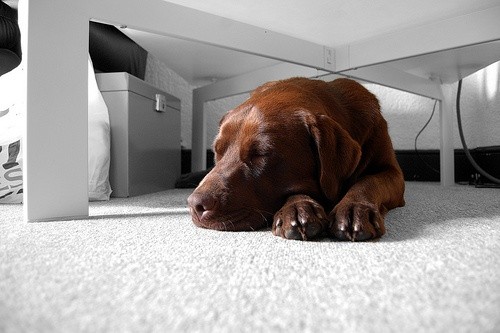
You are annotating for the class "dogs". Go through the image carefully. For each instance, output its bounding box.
[186,76,407,243]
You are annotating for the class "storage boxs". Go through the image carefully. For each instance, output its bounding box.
[95,72,181,199]
